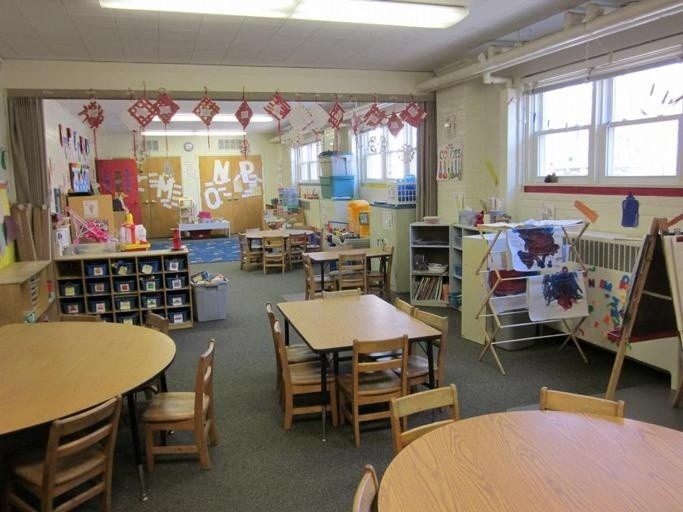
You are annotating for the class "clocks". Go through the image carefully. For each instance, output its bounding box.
[185,143,194,151]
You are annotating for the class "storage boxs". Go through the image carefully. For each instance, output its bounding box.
[493,312,537,350]
[319,154,354,199]
[54,245,195,331]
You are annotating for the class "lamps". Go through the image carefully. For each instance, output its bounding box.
[100,0,469,30]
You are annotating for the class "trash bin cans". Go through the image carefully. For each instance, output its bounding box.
[191,272,230,322]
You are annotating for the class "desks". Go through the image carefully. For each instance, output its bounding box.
[178,221,231,240]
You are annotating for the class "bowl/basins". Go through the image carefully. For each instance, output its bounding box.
[427,265,448,274]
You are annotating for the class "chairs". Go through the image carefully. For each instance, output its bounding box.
[239,227,394,299]
[266,287,449,449]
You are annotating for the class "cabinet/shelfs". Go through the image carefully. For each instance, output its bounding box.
[409,222,491,311]
[1,261,57,323]
[97,159,141,224]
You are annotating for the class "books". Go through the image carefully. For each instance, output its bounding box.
[414,276,443,300]
